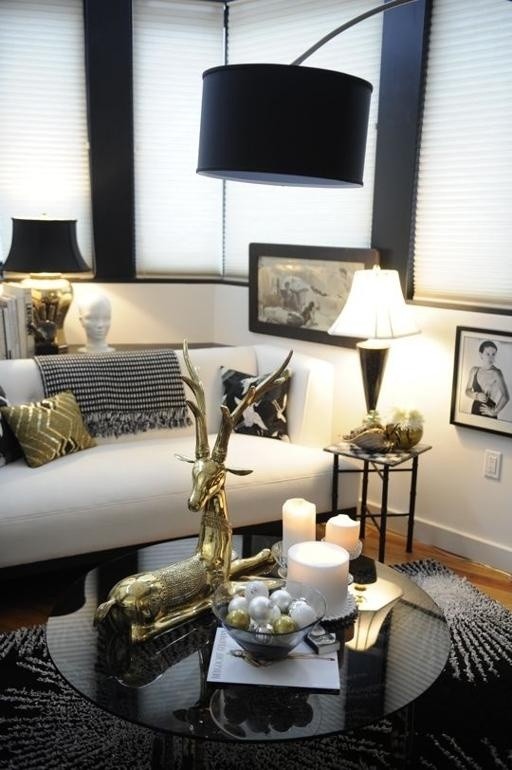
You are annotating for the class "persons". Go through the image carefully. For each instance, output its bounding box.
[465,341,509,419]
[77,293,112,353]
[229,649,335,670]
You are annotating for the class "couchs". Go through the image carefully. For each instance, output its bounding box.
[0,345,363,571]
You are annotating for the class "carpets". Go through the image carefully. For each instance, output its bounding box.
[0,558,512,770]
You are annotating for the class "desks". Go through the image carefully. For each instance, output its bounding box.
[323,440,433,564]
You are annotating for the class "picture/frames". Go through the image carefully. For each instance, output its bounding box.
[449,325,512,439]
[249,242,382,350]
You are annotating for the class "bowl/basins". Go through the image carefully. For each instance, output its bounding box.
[210,687,321,740]
[211,581,327,661]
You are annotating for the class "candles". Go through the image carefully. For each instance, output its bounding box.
[282,498,317,557]
[325,513,361,553]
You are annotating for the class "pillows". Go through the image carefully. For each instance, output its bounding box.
[0,387,25,468]
[218,365,294,446]
[0,388,98,468]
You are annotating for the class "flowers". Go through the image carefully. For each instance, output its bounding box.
[387,405,424,431]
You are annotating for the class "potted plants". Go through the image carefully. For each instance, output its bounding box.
[342,410,394,452]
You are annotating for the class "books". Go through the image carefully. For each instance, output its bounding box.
[0,277,34,361]
[207,627,343,692]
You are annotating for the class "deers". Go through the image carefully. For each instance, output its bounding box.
[94,337,292,646]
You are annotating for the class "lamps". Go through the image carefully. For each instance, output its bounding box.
[0,218,93,354]
[345,556,403,654]
[326,266,422,421]
[196,0,419,189]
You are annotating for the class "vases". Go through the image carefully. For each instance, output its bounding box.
[386,424,423,450]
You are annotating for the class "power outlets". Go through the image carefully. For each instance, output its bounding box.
[483,448,502,481]
